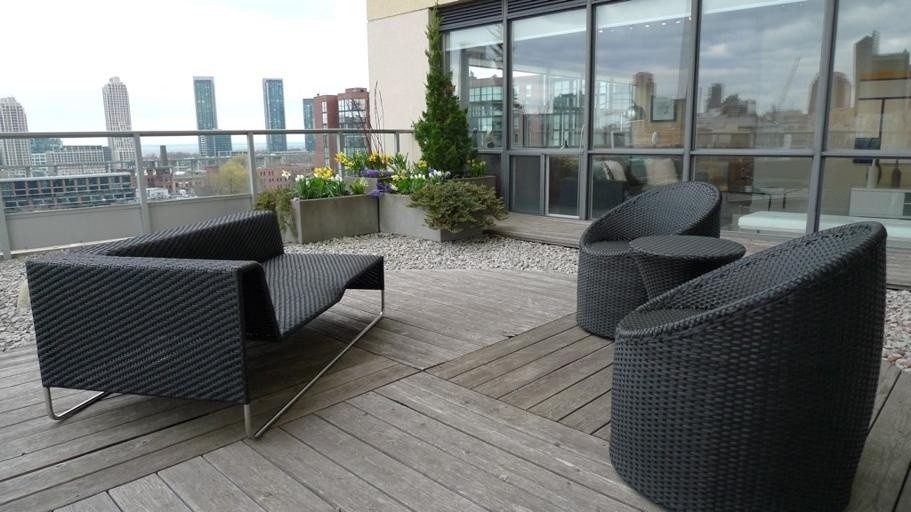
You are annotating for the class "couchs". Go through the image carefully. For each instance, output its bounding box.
[589,156,682,210]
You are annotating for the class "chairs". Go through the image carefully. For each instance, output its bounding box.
[607,220,887,511]
[577,181,721,339]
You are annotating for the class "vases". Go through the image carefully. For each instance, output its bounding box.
[375,186,483,245]
[341,173,378,197]
[865,156,902,188]
[456,173,498,197]
[283,191,380,243]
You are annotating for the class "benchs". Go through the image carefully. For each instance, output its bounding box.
[23,210,384,445]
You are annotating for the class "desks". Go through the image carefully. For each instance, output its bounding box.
[629,233,745,299]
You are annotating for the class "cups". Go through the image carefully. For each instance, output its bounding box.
[784,133,793,149]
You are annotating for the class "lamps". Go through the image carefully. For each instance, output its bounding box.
[596,98,646,124]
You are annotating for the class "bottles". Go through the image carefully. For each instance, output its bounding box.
[652,132,661,146]
[892,160,901,187]
[868,158,879,188]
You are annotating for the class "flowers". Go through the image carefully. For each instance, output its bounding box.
[254,165,362,235]
[411,155,487,179]
[364,160,450,200]
[332,152,409,175]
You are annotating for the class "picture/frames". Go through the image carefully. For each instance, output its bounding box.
[649,94,677,122]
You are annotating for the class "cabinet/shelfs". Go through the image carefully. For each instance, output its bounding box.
[847,187,911,220]
